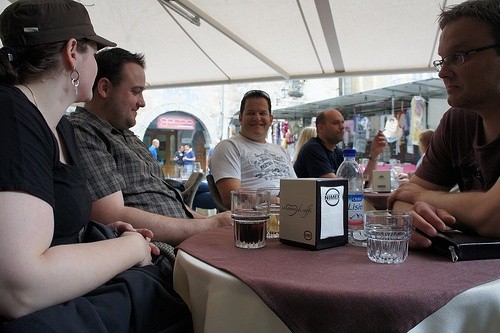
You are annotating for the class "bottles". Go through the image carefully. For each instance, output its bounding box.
[336,149,364,244]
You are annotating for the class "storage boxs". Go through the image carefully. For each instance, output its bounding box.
[280,177,348,250]
[373,169,395,193]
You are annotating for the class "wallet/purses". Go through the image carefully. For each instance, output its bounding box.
[408,226,500,263]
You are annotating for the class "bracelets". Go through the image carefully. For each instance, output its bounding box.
[369,154,380,160]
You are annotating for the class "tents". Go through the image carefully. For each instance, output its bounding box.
[0,0,500,90]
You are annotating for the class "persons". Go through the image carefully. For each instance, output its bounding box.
[149,139,159,159]
[210,90,298,209]
[67,48,233,273]
[398,130,434,180]
[182,143,195,179]
[387,0,500,248]
[293,110,387,189]
[0,0,192,333]
[174,143,192,178]
[295,127,317,162]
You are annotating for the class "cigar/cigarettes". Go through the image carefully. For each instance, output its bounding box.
[381,129,386,133]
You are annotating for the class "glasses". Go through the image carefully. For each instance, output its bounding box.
[244,90,270,99]
[433,44,500,72]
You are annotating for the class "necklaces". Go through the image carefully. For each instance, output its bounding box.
[21,83,37,106]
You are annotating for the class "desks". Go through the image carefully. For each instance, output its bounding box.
[364,189,397,210]
[173,224,500,333]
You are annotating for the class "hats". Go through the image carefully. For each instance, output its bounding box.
[0,0,117,47]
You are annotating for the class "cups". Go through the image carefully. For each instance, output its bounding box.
[364,210,412,265]
[257,187,281,239]
[230,190,266,249]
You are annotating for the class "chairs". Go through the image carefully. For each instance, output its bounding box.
[179,172,229,213]
